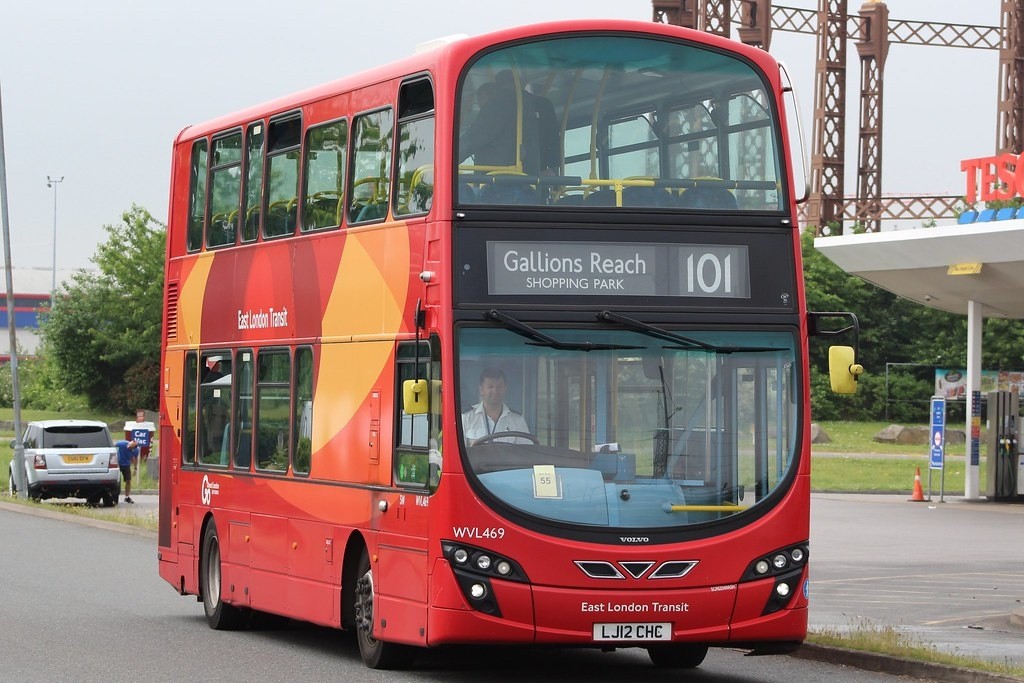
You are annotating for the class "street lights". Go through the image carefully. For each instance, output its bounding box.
[46,175,65,306]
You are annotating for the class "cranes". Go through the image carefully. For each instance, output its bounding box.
[652,1,1024,238]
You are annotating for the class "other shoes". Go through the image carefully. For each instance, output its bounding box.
[124,497,134,504]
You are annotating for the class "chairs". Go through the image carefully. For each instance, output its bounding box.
[220,423,276,466]
[207,164,739,246]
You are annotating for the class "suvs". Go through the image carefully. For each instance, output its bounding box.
[7,419,121,508]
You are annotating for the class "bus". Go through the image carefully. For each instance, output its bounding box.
[156,17,865,664]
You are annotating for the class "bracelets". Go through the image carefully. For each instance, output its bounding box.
[134,468,137,469]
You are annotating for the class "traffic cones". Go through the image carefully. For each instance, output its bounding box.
[907,466,930,502]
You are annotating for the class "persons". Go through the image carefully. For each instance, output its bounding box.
[458,82,540,193]
[462,368,533,445]
[114,440,139,503]
[496,70,562,202]
